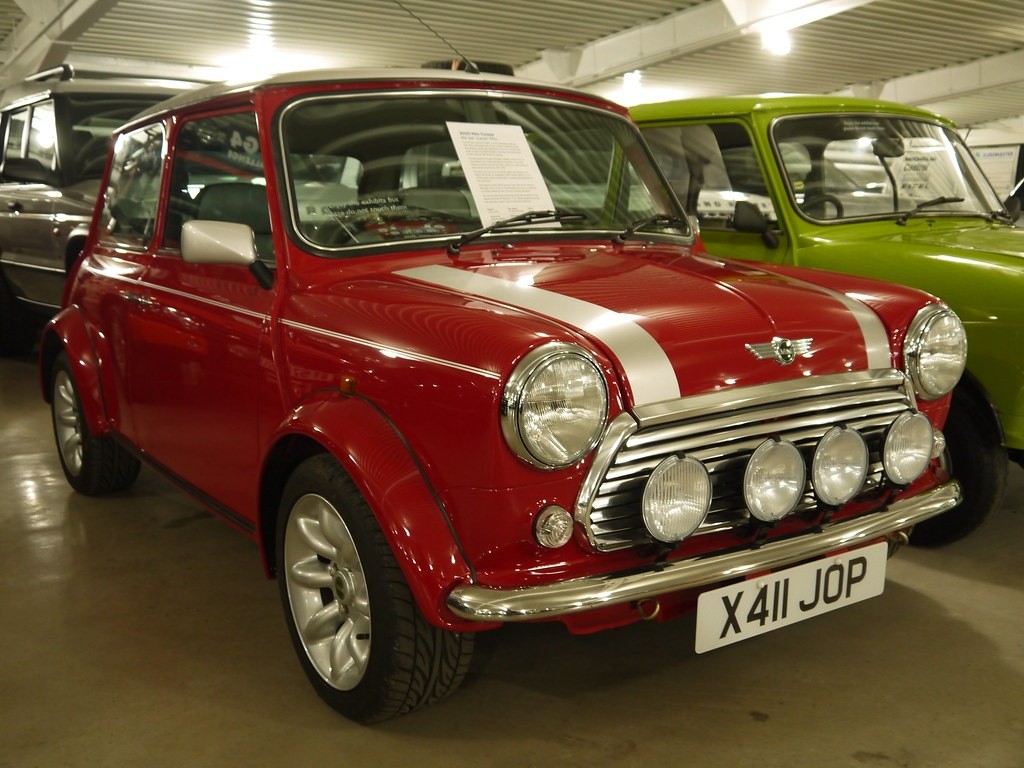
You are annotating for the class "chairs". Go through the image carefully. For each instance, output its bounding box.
[192,182,275,260]
[399,187,480,219]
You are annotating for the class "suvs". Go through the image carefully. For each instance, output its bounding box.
[0,67,226,367]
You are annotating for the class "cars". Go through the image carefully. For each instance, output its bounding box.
[565,95,1021,545]
[40,69,962,725]
